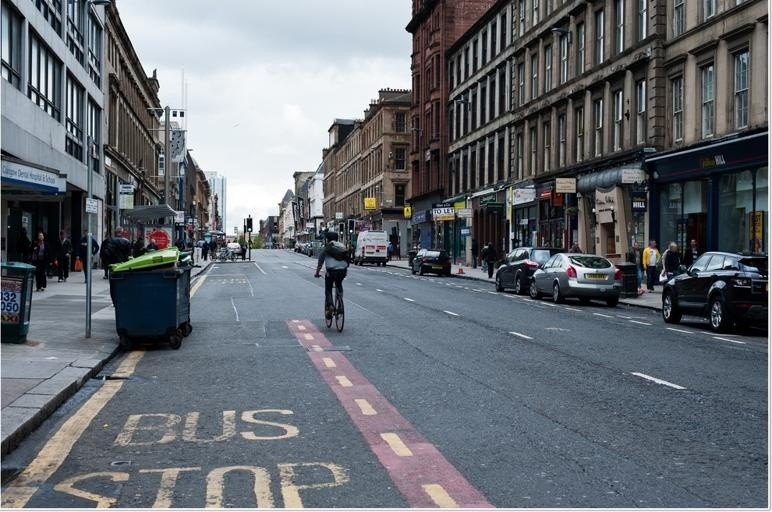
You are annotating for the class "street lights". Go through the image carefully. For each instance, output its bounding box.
[147,106,187,207]
[191,198,198,248]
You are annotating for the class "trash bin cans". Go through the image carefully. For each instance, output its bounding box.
[0,262,37,344]
[408,251,417,266]
[615,262,638,299]
[108,245,193,349]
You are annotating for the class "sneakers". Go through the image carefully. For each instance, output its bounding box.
[326,304,334,320]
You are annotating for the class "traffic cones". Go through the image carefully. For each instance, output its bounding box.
[456,262,466,275]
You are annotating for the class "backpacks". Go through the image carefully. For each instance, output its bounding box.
[325,240,349,261]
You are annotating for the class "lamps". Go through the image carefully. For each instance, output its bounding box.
[369,145,383,155]
[551,27,573,44]
[456,98,472,110]
[408,127,423,137]
[493,181,500,190]
[610,205,615,212]
[675,136,684,151]
[575,191,584,201]
[467,193,472,201]
[592,205,598,214]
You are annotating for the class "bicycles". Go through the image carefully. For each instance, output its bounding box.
[210,247,238,263]
[313,271,346,332]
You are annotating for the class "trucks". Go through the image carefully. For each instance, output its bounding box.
[353,230,389,267]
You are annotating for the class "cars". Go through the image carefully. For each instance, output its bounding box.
[294,241,325,257]
[226,242,242,255]
[412,248,451,276]
[528,252,623,306]
[662,251,767,333]
[495,247,568,295]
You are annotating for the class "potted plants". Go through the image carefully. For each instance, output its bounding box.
[566,207,579,217]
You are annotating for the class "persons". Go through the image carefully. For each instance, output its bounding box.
[388,242,401,260]
[628,239,701,295]
[315,230,349,312]
[571,242,582,253]
[481,242,497,278]
[28,225,248,291]
[413,242,421,251]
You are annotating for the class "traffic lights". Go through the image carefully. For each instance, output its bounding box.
[339,223,344,236]
[347,219,355,234]
[289,227,292,231]
[247,218,253,232]
